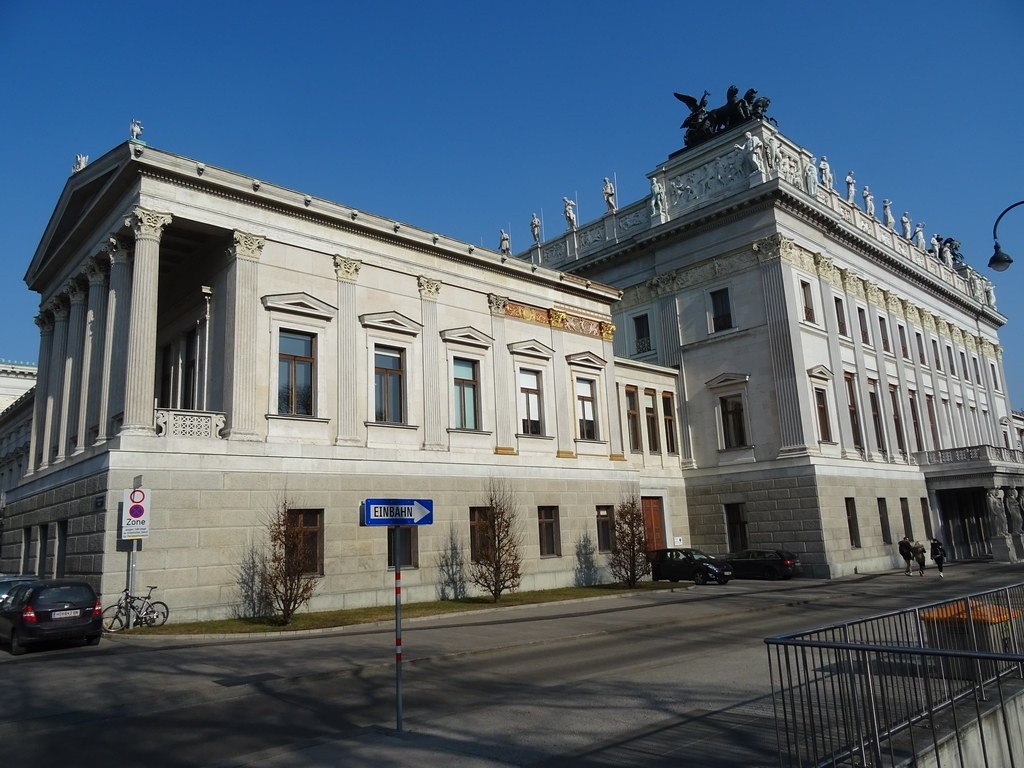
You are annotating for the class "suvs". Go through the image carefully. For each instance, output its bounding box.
[0,578,103,655]
[1,573,52,609]
[649,547,733,585]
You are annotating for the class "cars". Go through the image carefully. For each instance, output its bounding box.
[721,547,803,581]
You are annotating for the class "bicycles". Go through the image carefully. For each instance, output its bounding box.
[103,585,169,632]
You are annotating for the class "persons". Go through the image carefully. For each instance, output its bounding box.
[680,94,709,125]
[561,196,577,229]
[901,211,913,240]
[712,257,720,277]
[862,185,875,217]
[602,177,617,210]
[988,489,1024,536]
[735,127,834,197]
[649,178,663,216]
[971,274,998,307]
[845,169,857,203]
[497,229,511,253]
[914,222,928,251]
[883,198,897,229]
[930,231,955,270]
[896,533,946,579]
[529,213,542,244]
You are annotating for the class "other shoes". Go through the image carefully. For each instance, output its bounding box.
[919,570,925,577]
[939,572,943,578]
[910,572,912,576]
[905,572,909,576]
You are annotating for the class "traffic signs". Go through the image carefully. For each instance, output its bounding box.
[363,497,433,526]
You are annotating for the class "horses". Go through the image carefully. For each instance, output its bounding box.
[707,85,780,133]
